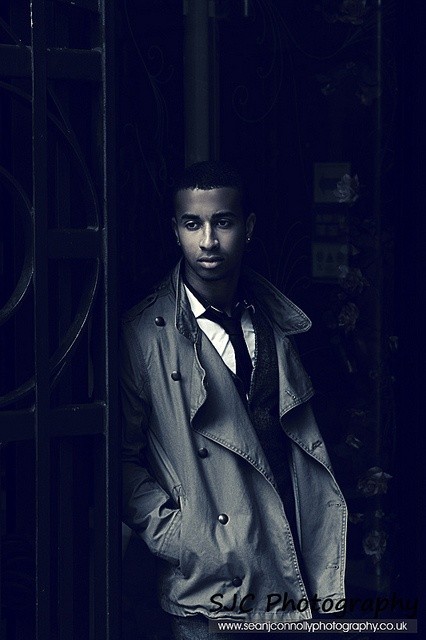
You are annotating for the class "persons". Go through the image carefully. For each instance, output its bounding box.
[113,164,345,630]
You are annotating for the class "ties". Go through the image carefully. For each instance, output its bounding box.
[203,304,253,394]
